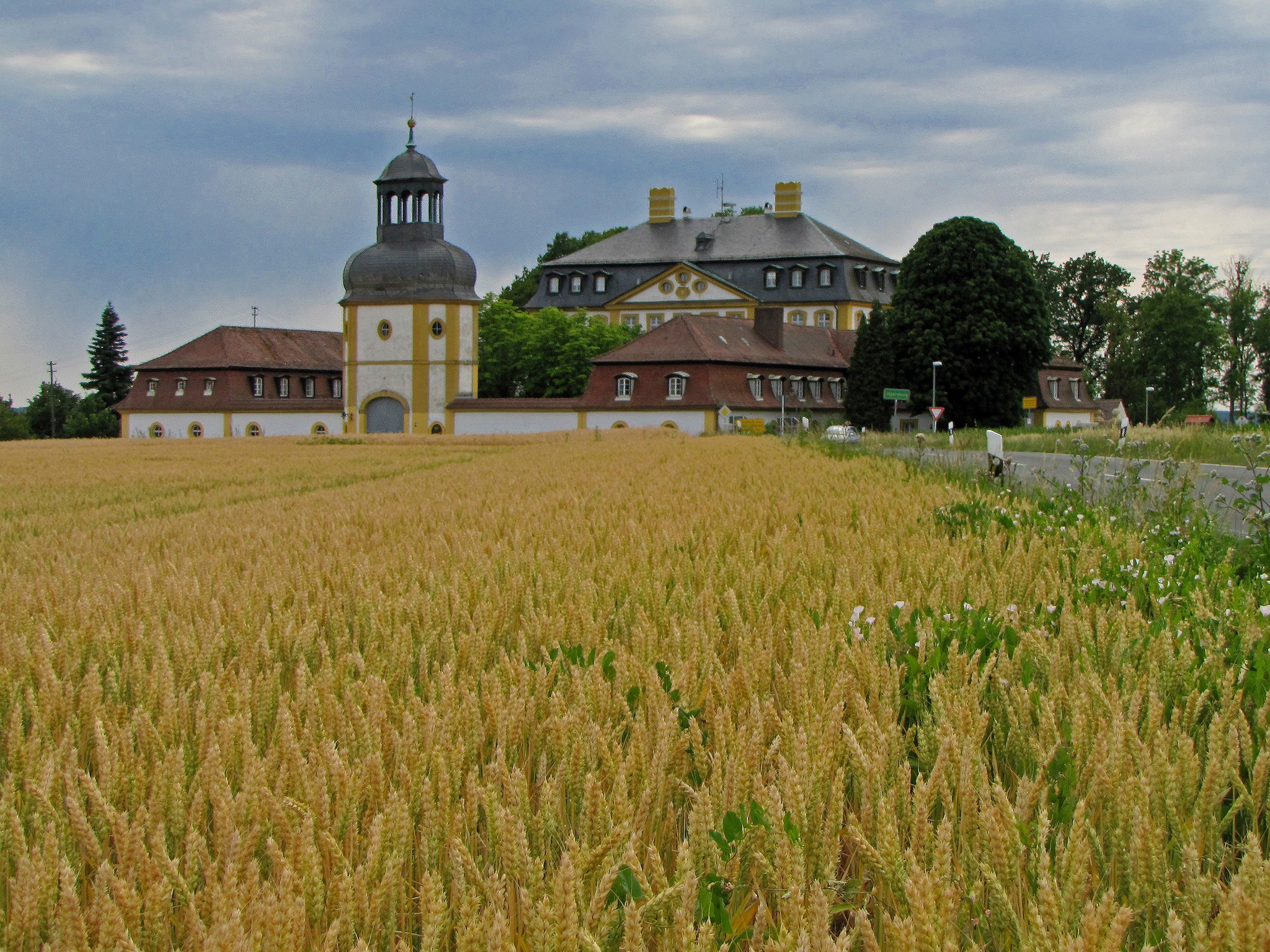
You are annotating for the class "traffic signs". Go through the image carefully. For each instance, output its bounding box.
[741,425,767,435]
[736,418,765,426]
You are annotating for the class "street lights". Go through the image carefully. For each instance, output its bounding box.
[1143,386,1155,430]
[932,361,943,432]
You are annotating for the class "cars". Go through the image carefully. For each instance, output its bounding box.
[825,426,860,443]
